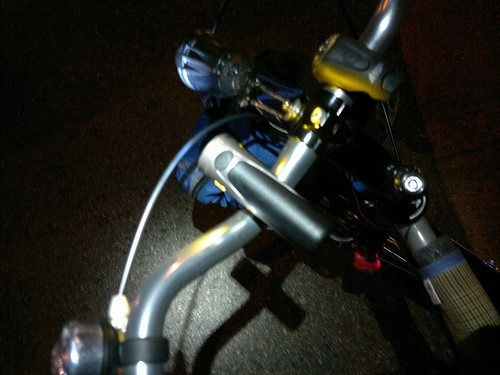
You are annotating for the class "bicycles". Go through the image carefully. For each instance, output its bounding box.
[50,0,500,375]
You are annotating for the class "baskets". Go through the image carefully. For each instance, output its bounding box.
[175,46,325,211]
